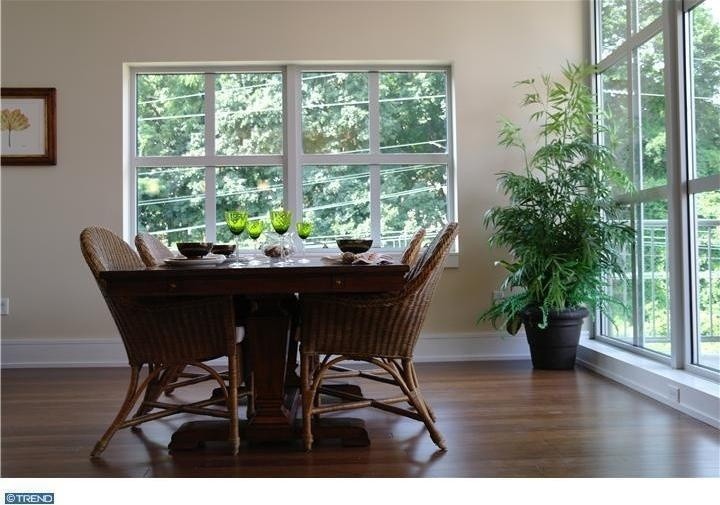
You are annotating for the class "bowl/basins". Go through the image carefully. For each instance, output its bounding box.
[177,243,213,259]
[210,245,236,257]
[336,240,373,254]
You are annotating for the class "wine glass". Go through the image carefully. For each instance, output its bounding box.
[224,211,312,268]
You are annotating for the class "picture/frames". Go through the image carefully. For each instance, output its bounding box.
[0,87,57,166]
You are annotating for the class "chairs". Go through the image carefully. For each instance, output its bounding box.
[475,57,639,371]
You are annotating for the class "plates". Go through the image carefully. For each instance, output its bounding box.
[164,254,225,267]
